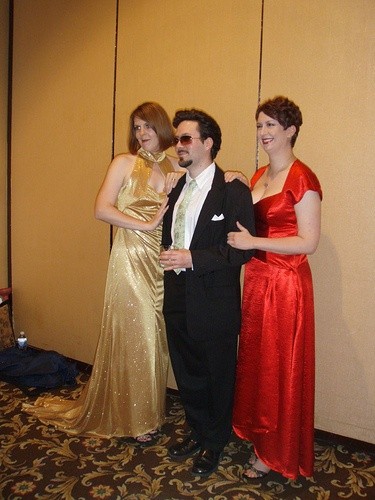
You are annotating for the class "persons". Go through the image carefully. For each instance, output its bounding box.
[159,108,255,476]
[19,95,322,480]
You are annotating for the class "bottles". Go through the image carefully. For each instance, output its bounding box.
[17,331,27,351]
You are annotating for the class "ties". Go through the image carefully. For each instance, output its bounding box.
[172,179,196,276]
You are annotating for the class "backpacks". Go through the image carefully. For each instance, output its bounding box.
[0,345,75,388]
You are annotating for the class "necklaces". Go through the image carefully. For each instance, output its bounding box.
[264,180,270,189]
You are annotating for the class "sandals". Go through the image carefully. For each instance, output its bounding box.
[241,449,274,481]
[122,427,157,446]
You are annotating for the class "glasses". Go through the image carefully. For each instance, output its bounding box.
[173,135,201,146]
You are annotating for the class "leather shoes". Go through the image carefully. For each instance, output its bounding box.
[167,437,200,457]
[191,444,224,476]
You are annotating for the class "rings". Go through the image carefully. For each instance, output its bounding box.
[172,178,175,181]
